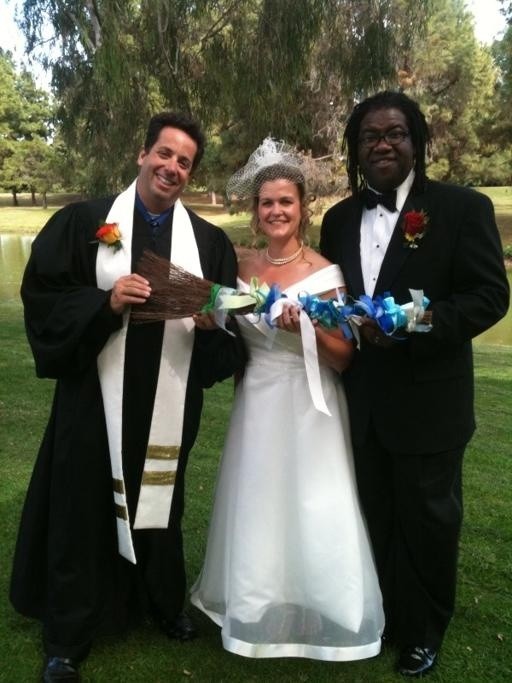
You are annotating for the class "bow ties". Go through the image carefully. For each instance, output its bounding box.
[364,188,396,212]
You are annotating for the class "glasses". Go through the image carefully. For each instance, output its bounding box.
[357,131,410,148]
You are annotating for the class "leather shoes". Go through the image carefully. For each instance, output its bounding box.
[43,656,79,683]
[396,646,439,676]
[158,610,196,641]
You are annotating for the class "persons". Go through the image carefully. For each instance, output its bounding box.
[8,111,238,683]
[318,90,510,677]
[189,162,386,662]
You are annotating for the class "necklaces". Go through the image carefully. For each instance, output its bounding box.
[264,238,304,265]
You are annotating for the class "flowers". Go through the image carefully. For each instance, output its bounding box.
[87,218,122,255]
[401,206,433,249]
